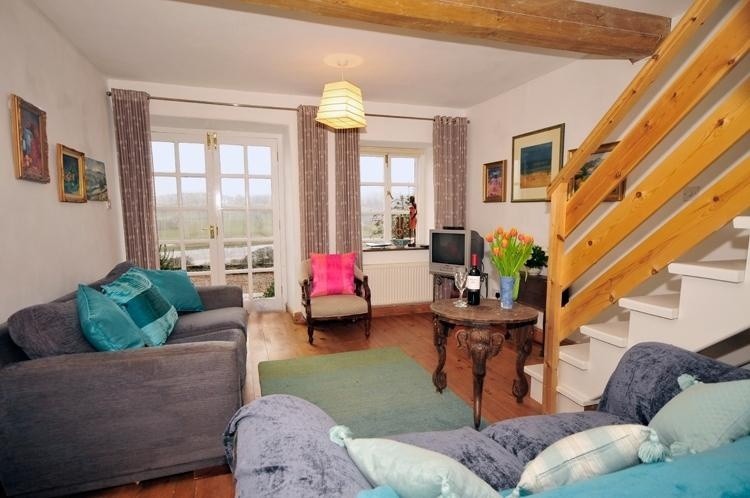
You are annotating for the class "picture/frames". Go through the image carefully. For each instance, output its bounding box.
[483,160,507,203]
[568,142,626,202]
[511,123,565,203]
[9,94,50,183]
[56,143,88,203]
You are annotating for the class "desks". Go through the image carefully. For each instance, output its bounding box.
[518,271,570,357]
[433,273,488,302]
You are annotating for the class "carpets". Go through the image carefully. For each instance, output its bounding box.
[258,347,488,438]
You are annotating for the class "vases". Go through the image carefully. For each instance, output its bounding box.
[499,276,515,309]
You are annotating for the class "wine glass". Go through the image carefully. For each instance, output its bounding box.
[453,270,468,308]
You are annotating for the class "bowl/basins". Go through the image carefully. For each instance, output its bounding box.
[390,238,412,249]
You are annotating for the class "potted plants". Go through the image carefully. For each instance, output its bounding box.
[524,246,549,276]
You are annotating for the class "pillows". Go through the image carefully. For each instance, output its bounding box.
[8,261,206,360]
[309,252,356,297]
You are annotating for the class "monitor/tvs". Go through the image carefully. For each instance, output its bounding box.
[429,229,484,275]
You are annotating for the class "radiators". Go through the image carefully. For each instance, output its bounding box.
[363,261,433,307]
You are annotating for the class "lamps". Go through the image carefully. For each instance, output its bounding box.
[315,53,367,130]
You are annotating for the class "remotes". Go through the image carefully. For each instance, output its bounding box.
[443,226,464,230]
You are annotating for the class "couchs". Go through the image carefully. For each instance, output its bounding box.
[223,341,750,498]
[0,284,248,498]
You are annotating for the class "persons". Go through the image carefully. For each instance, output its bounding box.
[408,195,418,247]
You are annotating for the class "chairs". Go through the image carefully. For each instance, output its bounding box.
[298,259,371,345]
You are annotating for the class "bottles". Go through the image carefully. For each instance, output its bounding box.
[466,253,481,305]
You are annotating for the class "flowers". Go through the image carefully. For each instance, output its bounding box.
[485,225,534,277]
